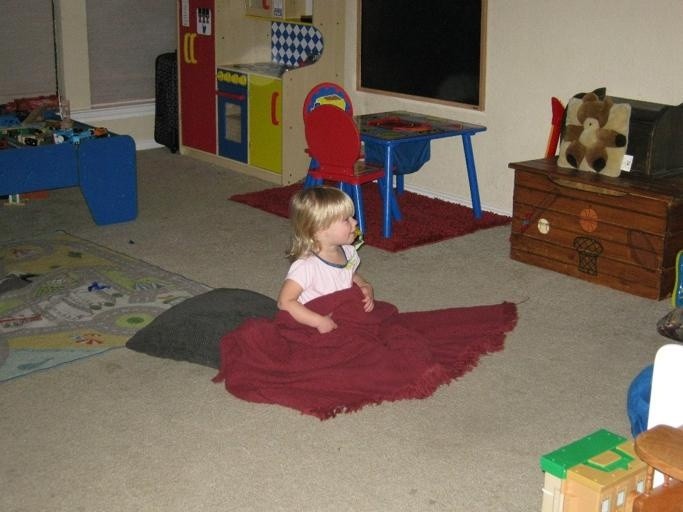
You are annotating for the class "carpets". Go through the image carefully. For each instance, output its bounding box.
[1,227,215,384]
[228,176,511,255]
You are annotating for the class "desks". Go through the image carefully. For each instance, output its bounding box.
[352,110,488,238]
[0,111,139,225]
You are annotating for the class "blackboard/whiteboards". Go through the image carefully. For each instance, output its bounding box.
[356,0,488,112]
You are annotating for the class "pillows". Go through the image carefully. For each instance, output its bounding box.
[124,287,280,369]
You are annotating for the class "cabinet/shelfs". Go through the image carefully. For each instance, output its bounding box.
[175,0,346,187]
[506,156,683,302]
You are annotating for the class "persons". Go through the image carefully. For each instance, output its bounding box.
[275,186,376,335]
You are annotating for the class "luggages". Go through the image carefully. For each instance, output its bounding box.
[154,47,179,154]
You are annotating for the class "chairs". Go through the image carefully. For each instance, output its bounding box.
[302,80,402,236]
